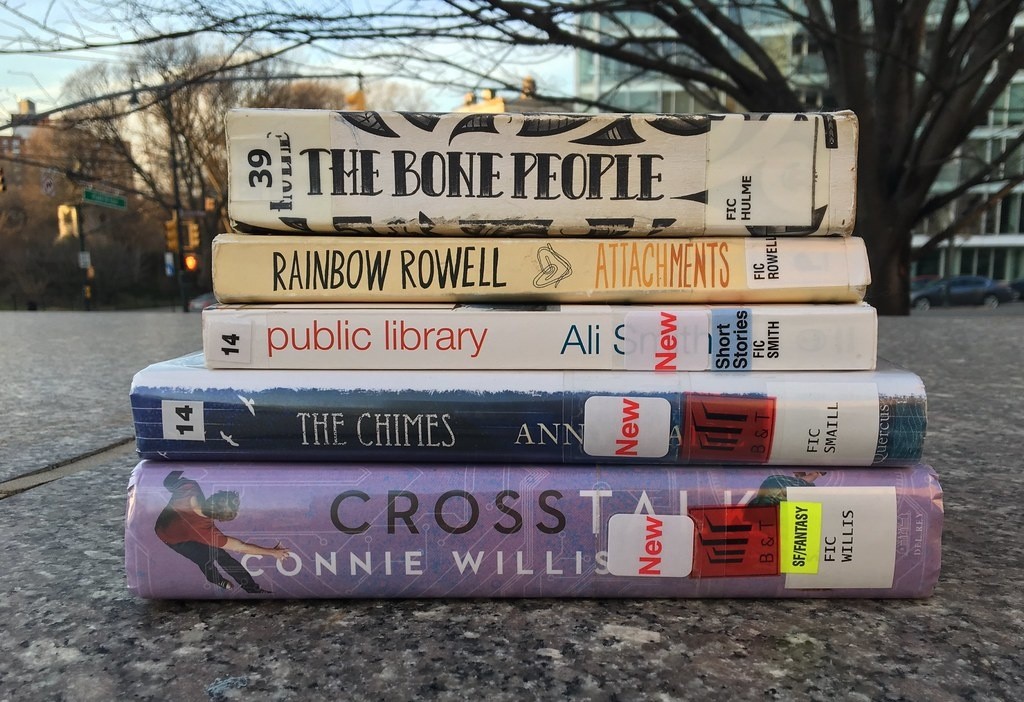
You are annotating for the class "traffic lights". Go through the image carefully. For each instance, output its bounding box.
[0,168,7,191]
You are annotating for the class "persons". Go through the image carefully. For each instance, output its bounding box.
[154,480,291,593]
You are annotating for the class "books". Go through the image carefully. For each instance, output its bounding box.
[123,108,945,598]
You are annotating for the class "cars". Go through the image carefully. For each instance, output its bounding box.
[1008,278,1024,302]
[188,292,218,312]
[909,275,1012,311]
[909,275,940,291]
